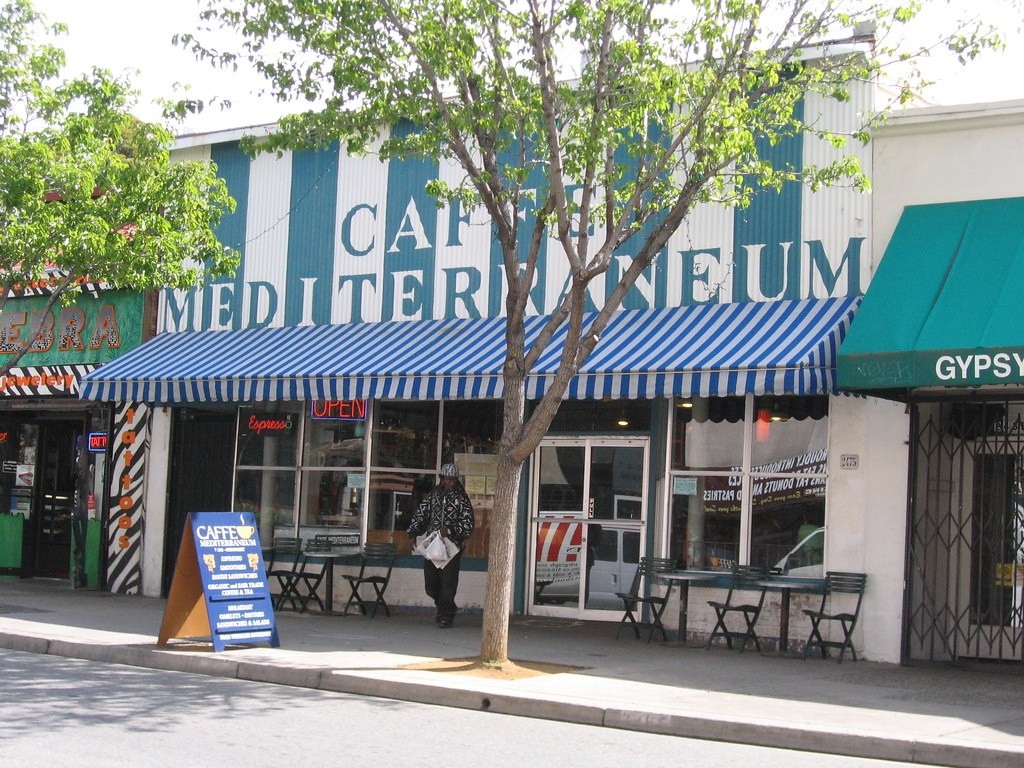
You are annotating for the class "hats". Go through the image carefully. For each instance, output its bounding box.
[439,463,459,478]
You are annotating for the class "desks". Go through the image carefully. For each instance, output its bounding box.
[303,550,360,617]
[755,581,822,659]
[657,572,716,648]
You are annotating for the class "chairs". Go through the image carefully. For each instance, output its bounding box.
[706,564,784,654]
[261,537,398,620]
[615,556,685,648]
[802,572,867,664]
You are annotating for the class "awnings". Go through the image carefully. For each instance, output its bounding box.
[77,293,870,405]
[835,196,1024,391]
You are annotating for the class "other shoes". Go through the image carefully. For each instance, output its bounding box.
[436,612,454,628]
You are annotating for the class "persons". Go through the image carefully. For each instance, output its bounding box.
[407,463,475,627]
